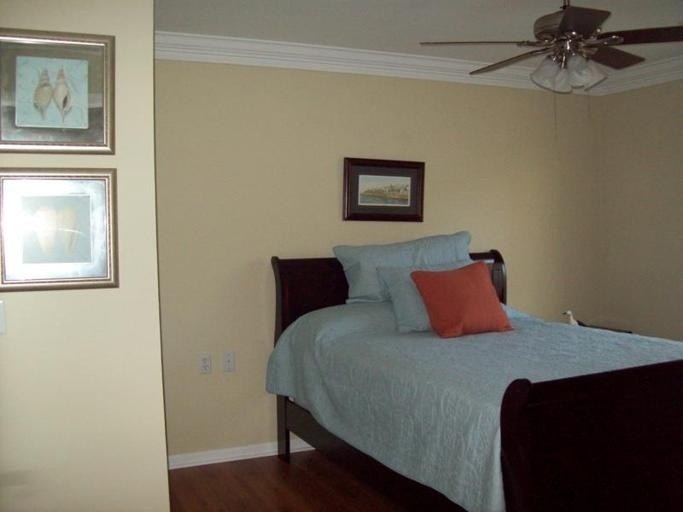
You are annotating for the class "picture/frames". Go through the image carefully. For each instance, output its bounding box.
[0,25,117,156]
[341,155,428,224]
[0,165,121,293]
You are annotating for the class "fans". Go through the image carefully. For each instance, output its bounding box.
[414,1,683,76]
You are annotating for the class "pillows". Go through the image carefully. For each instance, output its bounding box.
[410,259,517,340]
[375,257,475,335]
[331,227,476,305]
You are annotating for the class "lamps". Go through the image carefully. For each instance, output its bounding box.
[529,31,608,94]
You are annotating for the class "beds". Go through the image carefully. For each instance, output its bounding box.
[264,248,682,511]
